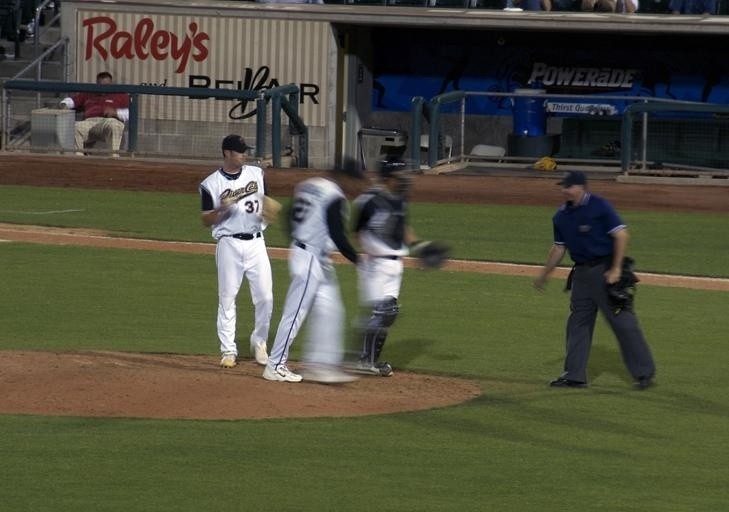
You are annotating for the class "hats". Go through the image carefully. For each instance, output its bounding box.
[222,135,254,153]
[555,171,585,184]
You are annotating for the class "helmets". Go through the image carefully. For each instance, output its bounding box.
[609,269,640,302]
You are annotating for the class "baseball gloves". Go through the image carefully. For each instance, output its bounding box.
[409,240,449,270]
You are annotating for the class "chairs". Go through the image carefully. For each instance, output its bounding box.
[465,143,506,164]
[419,134,454,164]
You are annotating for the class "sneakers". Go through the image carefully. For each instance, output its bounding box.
[250,334,269,366]
[551,372,588,388]
[633,372,656,386]
[262,365,303,383]
[353,362,394,376]
[220,353,237,368]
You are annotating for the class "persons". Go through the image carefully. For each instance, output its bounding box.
[500,0,729,16]
[196,133,273,367]
[531,172,656,389]
[354,158,450,375]
[263,157,371,382]
[59,72,131,156]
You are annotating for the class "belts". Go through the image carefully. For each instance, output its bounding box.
[295,242,326,256]
[387,256,400,260]
[233,233,260,239]
